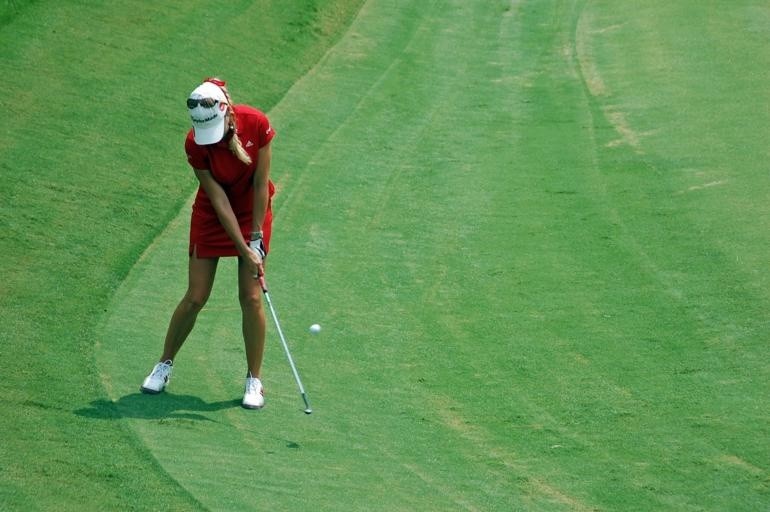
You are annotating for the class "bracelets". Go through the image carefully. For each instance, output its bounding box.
[251,230,263,239]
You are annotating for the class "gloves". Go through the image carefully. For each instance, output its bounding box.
[247,237,267,259]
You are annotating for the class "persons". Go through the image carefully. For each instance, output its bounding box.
[139,78,277,409]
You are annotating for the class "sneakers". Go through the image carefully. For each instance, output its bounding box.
[240,376,264,410]
[139,358,174,394]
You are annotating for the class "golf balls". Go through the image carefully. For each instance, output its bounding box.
[310,324,321,335]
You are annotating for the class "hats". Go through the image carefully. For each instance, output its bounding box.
[186,82,229,146]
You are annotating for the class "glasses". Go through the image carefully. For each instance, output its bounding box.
[186,98,218,109]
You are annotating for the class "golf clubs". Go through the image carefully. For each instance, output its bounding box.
[259,274,313,415]
